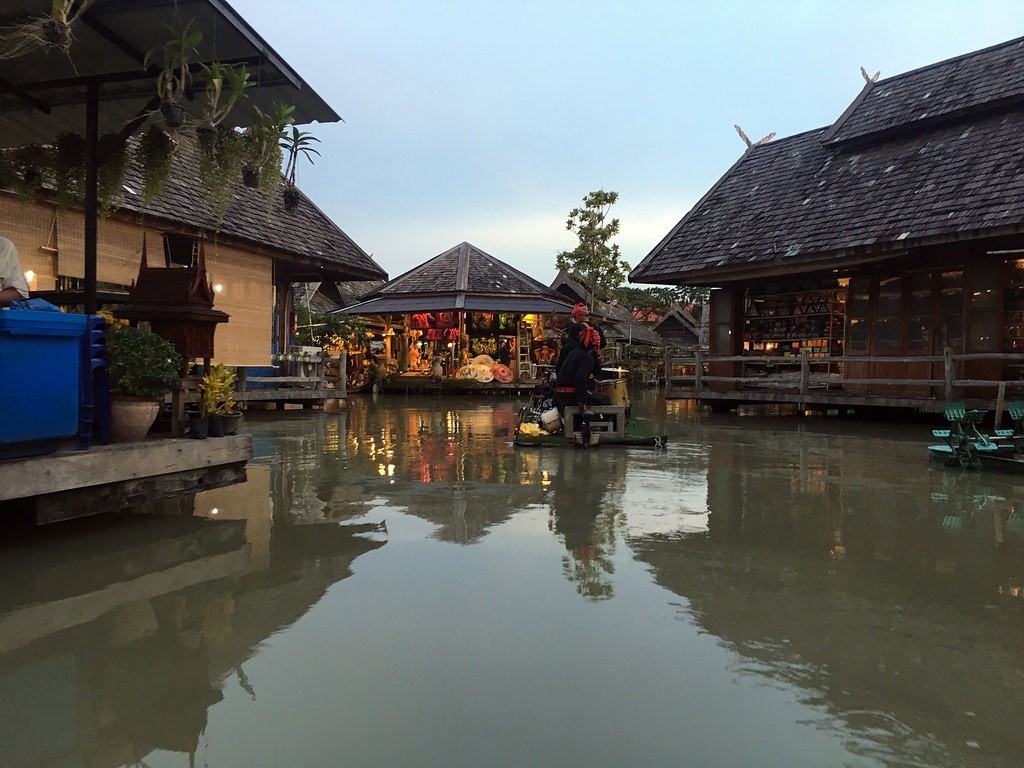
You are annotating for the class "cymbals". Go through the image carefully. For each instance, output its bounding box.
[602,346,621,352]
[600,367,629,372]
[532,365,555,368]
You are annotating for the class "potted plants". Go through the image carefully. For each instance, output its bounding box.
[199,362,236,437]
[0,0,323,274]
[103,325,184,444]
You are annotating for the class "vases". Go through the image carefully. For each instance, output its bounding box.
[224,410,243,434]
[187,410,209,438]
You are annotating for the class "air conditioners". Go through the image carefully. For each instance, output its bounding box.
[288,346,318,388]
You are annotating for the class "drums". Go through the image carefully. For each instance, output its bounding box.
[540,406,564,434]
[598,378,629,405]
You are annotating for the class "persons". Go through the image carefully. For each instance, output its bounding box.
[408,302,617,430]
[0,236,29,310]
